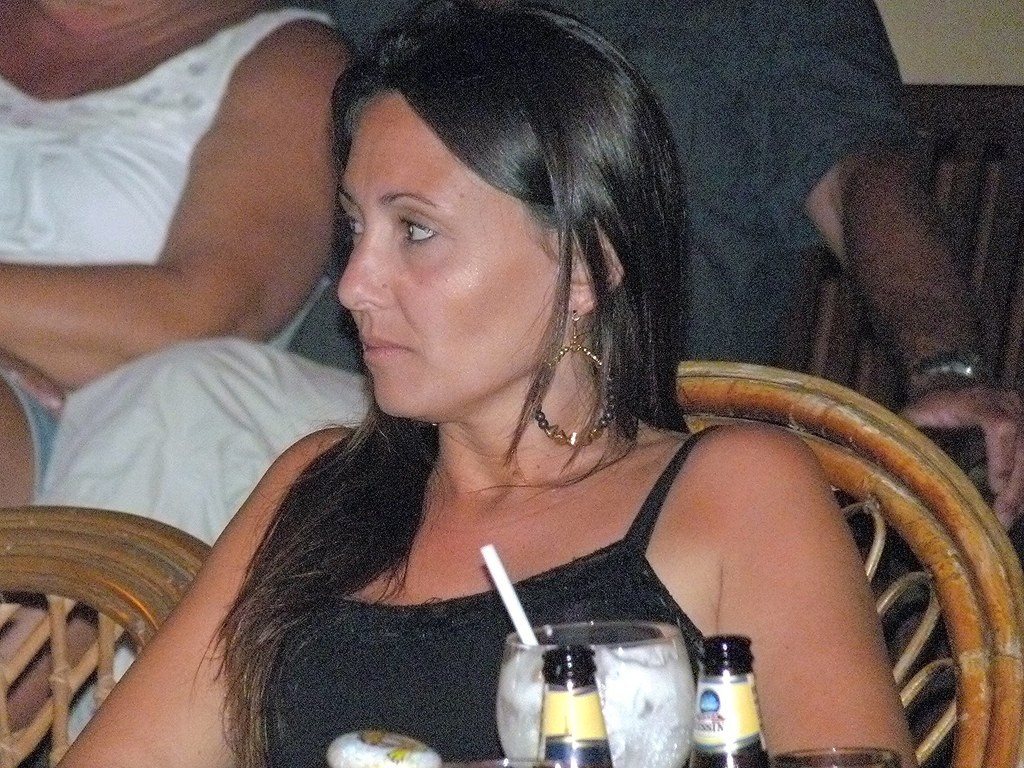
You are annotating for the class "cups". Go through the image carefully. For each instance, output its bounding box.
[495,620,695,768]
[770,746,905,768]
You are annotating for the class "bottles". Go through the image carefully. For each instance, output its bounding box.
[537,644,614,767]
[688,633,770,768]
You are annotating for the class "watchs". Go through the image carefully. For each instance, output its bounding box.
[907,352,996,387]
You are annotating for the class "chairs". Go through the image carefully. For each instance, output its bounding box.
[0,503,208,768]
[674,357,1024,766]
[808,87,1024,554]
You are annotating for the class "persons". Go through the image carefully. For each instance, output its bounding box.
[0,0,353,510]
[55,0,1024,768]
